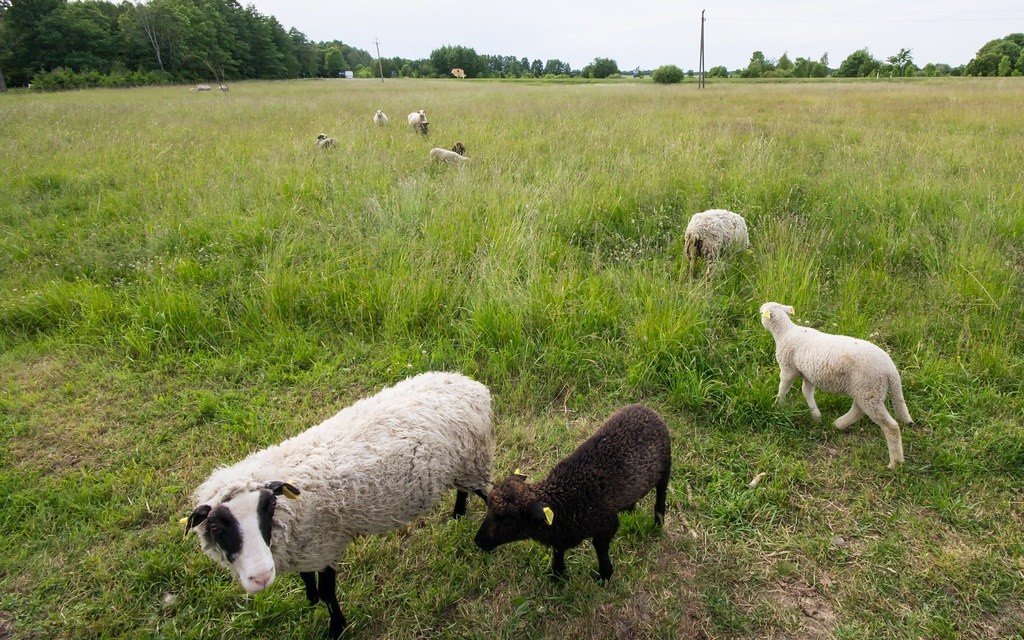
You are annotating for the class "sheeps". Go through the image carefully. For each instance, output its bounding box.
[682,208,752,289]
[181,371,495,639]
[473,404,672,582]
[759,301,915,472]
[316,109,471,167]
